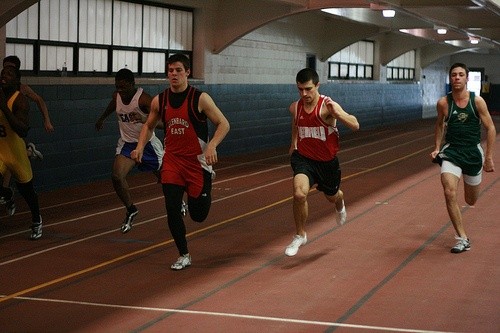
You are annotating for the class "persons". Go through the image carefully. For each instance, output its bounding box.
[480,74,492,111]
[429,63,496,253]
[283,68,359,256]
[0,74,43,240]
[0,55,55,217]
[94,68,165,234]
[131,53,231,270]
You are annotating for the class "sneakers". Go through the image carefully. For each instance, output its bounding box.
[171,253,191,270]
[335,200,346,225]
[121,205,138,233]
[450,234,471,253]
[0,183,16,216]
[180,200,189,216]
[285,232,307,257]
[25,143,44,162]
[29,214,42,241]
